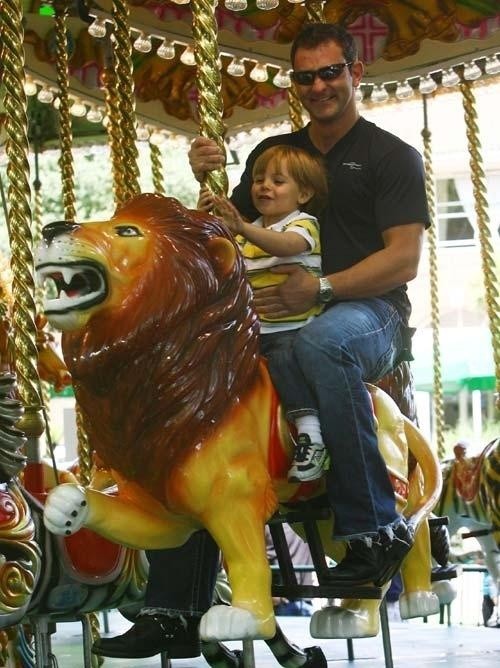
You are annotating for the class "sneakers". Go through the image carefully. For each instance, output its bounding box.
[287,437,331,482]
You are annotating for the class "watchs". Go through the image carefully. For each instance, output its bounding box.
[318,277,332,306]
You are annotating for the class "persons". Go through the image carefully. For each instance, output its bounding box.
[91,23,427,657]
[90,23,432,659]
[192,146,332,484]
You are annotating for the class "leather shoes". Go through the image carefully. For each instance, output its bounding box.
[90,617,201,659]
[325,519,415,583]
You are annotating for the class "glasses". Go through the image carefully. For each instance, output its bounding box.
[292,62,353,83]
